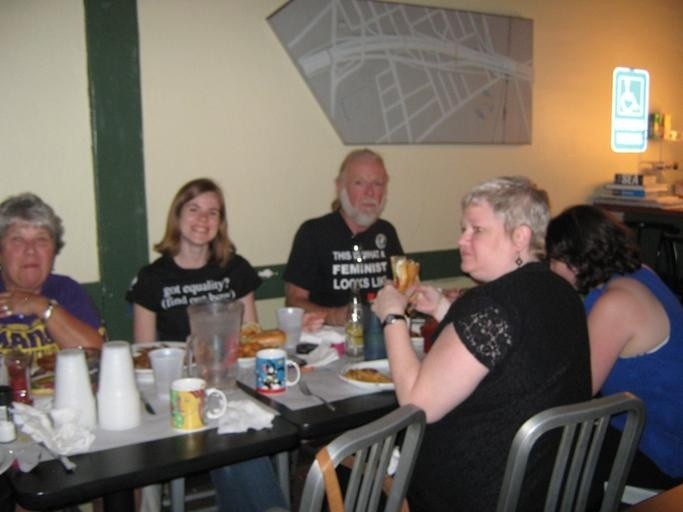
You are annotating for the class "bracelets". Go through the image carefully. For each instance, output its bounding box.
[40,299,57,323]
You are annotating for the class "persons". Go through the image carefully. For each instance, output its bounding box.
[282,148,414,512]
[544,203,683,491]
[370,177,610,512]
[1,192,136,511]
[123,178,292,512]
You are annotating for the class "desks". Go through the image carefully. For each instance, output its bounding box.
[594,199,683,277]
[627,484,683,511]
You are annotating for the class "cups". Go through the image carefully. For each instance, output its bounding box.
[271,307,306,353]
[147,348,186,400]
[50,340,140,432]
[256,349,308,396]
[171,378,227,433]
[186,300,245,388]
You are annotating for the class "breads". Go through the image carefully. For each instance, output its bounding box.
[241,329,284,356]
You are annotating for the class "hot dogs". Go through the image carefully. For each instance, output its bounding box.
[394,258,420,315]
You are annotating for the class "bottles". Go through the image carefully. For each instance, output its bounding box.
[0,344,32,442]
[366,291,385,358]
[346,277,363,359]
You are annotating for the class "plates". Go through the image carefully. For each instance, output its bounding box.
[410,317,428,348]
[235,356,256,365]
[340,358,396,390]
[33,345,98,391]
[129,340,186,375]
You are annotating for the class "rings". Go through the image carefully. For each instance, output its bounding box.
[2,305,9,311]
[7,311,13,316]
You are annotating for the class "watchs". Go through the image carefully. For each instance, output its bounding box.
[380,313,407,329]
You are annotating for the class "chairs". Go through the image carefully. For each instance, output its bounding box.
[620,217,679,282]
[492,389,646,510]
[297,402,428,512]
[659,229,682,298]
